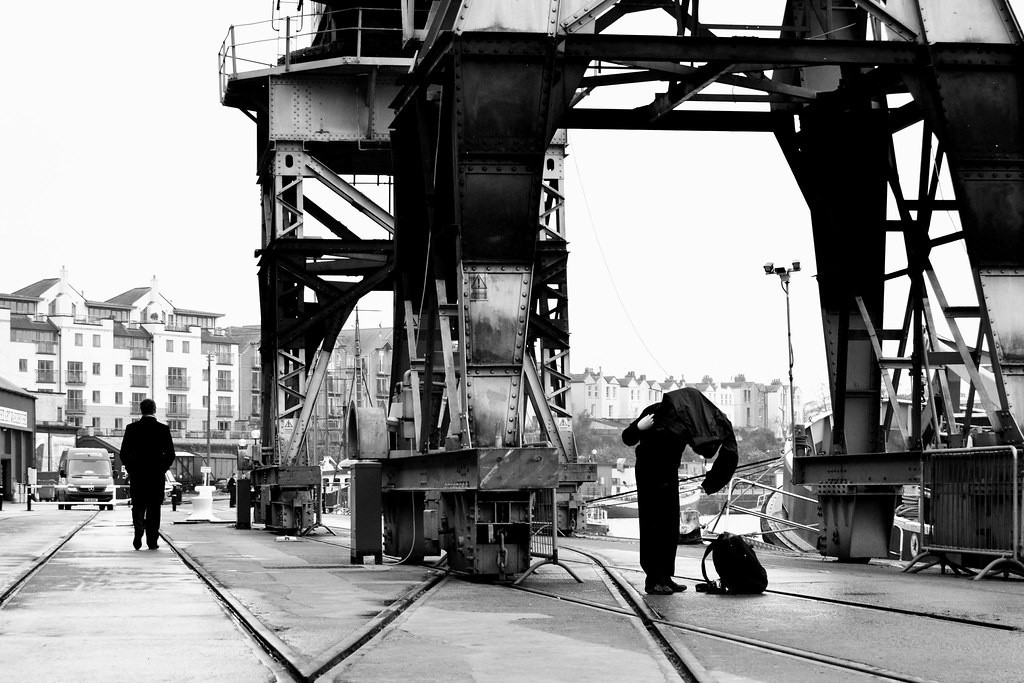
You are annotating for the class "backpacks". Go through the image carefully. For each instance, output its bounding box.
[702,532,768,595]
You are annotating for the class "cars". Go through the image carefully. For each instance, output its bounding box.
[217,478,230,491]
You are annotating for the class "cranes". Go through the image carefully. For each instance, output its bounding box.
[332,304,386,484]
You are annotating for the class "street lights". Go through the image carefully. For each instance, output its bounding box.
[205,353,216,484]
[760,257,804,462]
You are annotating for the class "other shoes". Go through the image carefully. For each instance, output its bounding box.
[133,531,142,550]
[645,582,686,595]
[230,505,235,508]
[149,545,159,549]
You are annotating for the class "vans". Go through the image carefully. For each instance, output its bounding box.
[55,446,116,511]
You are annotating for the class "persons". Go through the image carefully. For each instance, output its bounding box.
[621,387,728,595]
[227,473,236,508]
[119,399,176,550]
